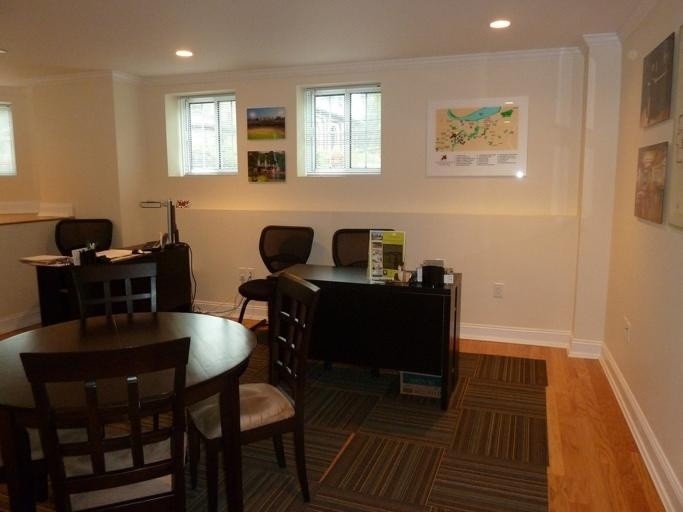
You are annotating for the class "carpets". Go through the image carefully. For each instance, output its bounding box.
[0,329,549,511]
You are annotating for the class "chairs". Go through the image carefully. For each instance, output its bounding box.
[17,335,192,512]
[238,226,396,325]
[54,218,112,255]
[68,263,160,318]
[187,271,320,510]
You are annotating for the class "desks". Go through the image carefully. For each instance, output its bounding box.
[17,241,191,327]
[1,310,258,510]
[266,264,463,412]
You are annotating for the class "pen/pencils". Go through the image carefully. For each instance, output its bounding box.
[88,239,96,250]
